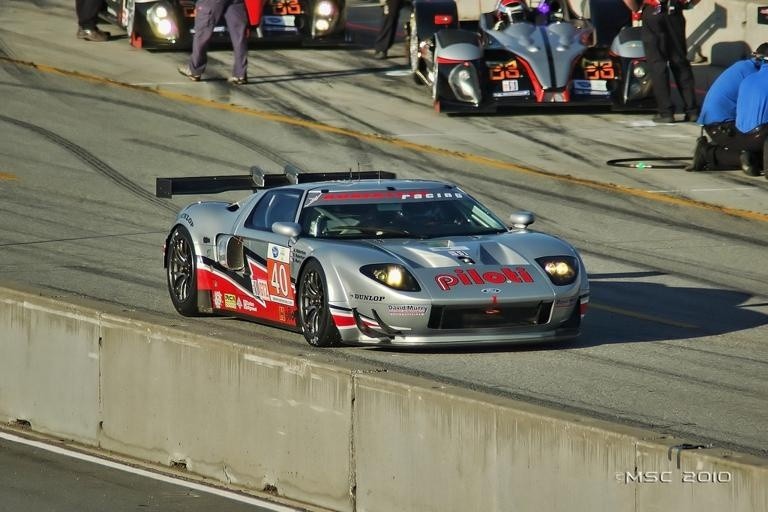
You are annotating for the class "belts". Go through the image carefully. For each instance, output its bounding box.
[748,124,765,136]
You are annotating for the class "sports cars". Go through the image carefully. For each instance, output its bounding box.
[153,165,588,349]
[405,0,662,116]
[95,0,346,50]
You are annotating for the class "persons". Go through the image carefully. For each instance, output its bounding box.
[75,0,111,42]
[625,0,703,124]
[178,1,248,85]
[734,52,767,178]
[371,0,417,61]
[686,43,767,172]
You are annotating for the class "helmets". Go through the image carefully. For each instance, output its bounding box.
[496,0,529,24]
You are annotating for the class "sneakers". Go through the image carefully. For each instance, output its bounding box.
[739,149,761,177]
[375,51,386,60]
[176,63,201,81]
[685,112,698,122]
[228,76,247,86]
[76,27,111,42]
[652,116,676,124]
[694,136,709,172]
